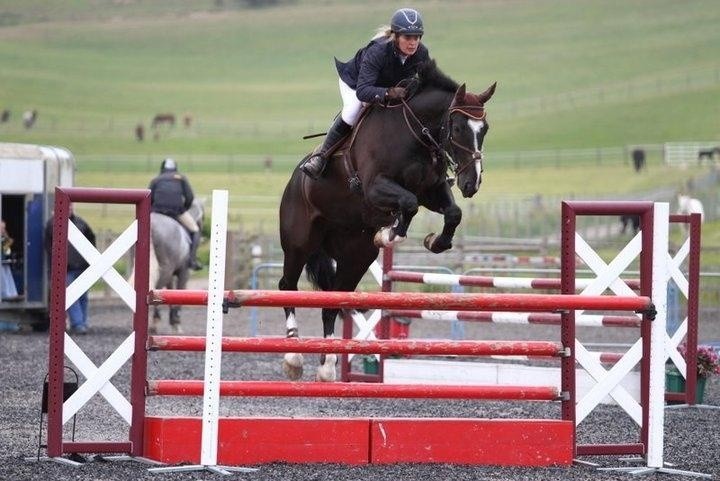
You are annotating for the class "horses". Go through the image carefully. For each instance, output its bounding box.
[127,195,209,335]
[277,57,501,387]
[673,191,705,233]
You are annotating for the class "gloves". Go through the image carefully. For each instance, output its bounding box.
[388,87,407,100]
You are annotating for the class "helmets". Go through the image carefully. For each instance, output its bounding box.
[390,8,424,36]
[161,159,176,171]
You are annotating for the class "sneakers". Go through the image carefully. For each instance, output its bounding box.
[75,324,87,334]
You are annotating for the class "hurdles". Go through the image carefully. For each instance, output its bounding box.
[46,185,700,470]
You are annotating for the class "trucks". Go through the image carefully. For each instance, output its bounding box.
[0,142,75,331]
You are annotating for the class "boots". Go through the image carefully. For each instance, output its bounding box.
[303,113,354,177]
[188,232,202,270]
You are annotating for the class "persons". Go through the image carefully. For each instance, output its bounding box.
[43,202,95,336]
[146,158,204,270]
[299,5,432,182]
